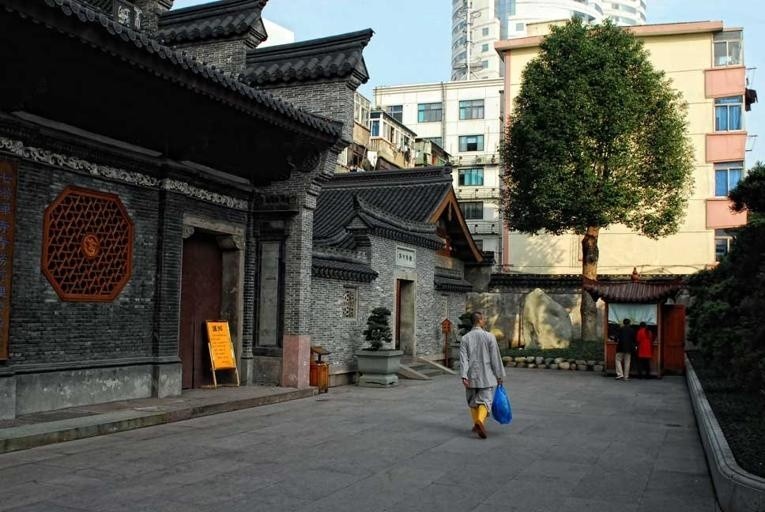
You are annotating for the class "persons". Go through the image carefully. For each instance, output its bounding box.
[615,319,636,380]
[634,322,655,379]
[459,312,507,440]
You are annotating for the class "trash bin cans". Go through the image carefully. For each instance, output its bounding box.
[310,346,331,394]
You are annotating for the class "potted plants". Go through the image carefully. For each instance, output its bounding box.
[450,312,473,370]
[354,307,404,389]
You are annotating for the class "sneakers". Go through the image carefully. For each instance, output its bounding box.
[473,420,487,438]
[615,375,628,380]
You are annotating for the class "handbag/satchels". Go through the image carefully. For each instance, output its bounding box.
[493,387,512,424]
